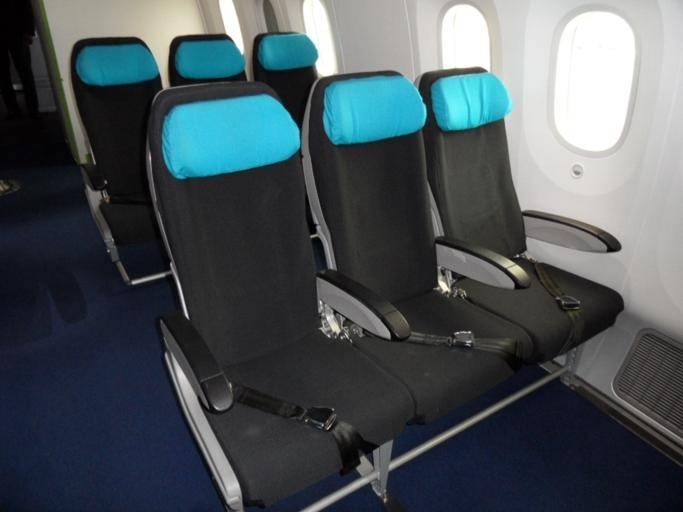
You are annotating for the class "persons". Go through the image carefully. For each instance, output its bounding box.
[0,1,41,123]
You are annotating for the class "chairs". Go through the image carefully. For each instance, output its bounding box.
[301,71,536,428]
[146,81,416,511]
[251,33,319,128]
[69,36,165,286]
[168,33,247,87]
[414,65,624,392]
